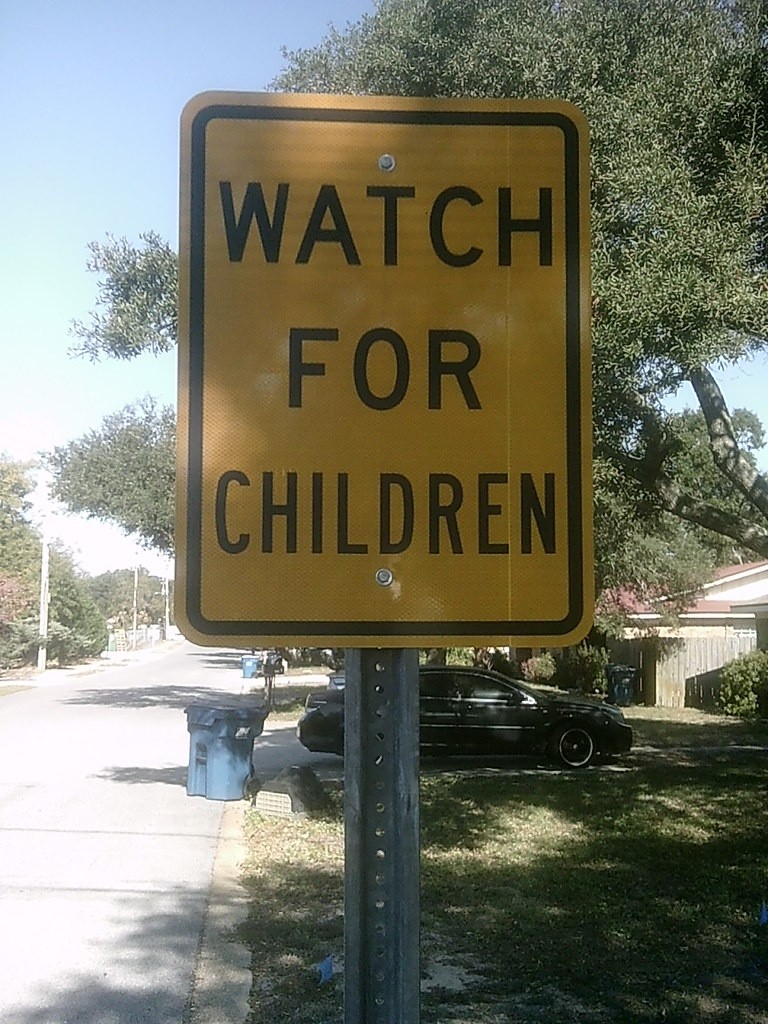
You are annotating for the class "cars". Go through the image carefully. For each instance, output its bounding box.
[298,663,634,771]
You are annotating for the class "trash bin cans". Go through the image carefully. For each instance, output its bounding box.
[604,663,638,707]
[184,699,273,800]
